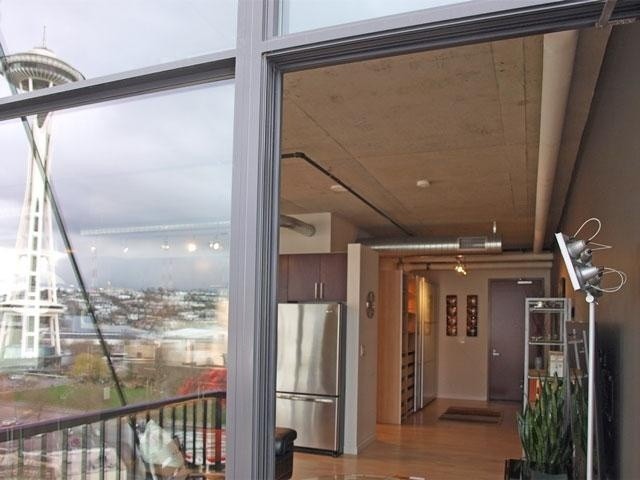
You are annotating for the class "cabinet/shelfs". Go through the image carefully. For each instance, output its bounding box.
[377,269,418,425]
[523,296,572,415]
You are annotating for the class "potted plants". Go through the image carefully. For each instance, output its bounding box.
[504,372,578,480]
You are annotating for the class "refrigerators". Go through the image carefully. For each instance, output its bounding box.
[276,300,346,455]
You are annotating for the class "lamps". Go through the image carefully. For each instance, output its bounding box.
[453,254,467,278]
[556,215,629,479]
